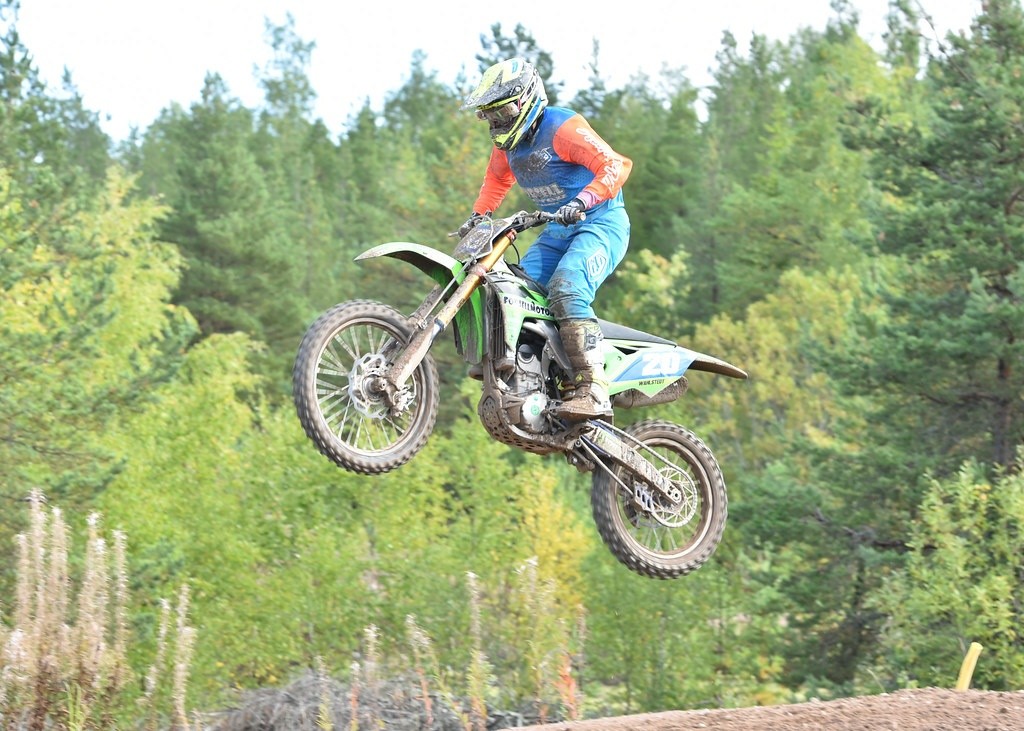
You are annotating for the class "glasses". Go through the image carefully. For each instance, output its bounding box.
[485,98,521,129]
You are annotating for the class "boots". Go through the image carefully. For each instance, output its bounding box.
[541,319,614,420]
[468,364,483,381]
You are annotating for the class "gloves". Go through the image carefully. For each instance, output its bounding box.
[554,198,585,227]
[457,213,486,240]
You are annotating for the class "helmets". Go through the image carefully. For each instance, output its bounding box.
[465,58,549,151]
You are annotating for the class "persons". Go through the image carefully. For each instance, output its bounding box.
[458,57,634,419]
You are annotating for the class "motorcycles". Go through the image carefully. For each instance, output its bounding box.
[292,205,750,583]
